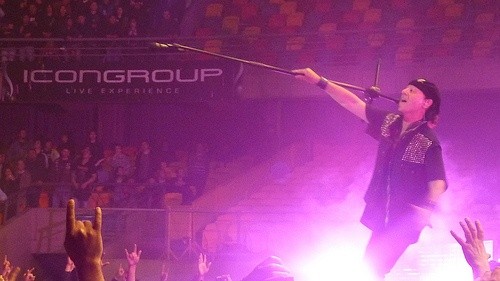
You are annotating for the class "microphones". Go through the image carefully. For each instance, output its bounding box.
[149,43,186,52]
